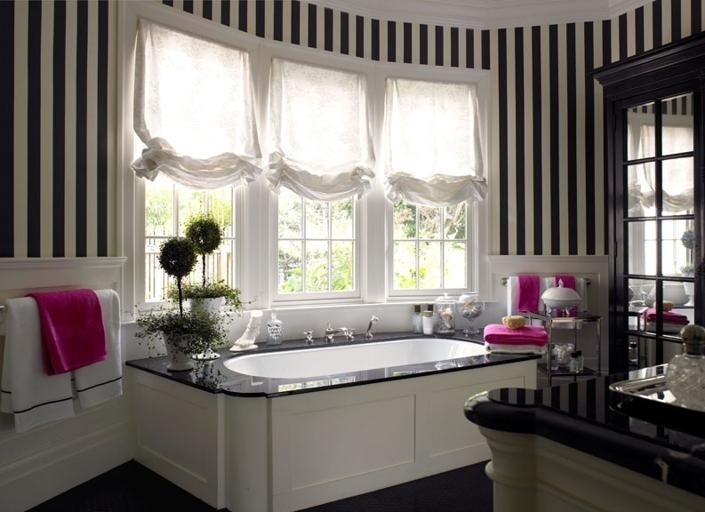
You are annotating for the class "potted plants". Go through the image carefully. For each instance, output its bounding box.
[163,199,256,361]
[121,234,227,392]
[680,230,695,307]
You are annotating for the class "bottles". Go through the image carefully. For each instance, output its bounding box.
[569,349,584,372]
[628,341,638,362]
[547,343,558,370]
[665,324,705,411]
[433,292,487,333]
[412,303,436,335]
[267,312,283,344]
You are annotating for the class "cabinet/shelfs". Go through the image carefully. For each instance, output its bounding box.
[523,313,603,388]
[628,304,648,373]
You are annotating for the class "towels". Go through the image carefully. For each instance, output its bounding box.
[0,296,76,434]
[73,289,124,409]
[517,275,540,313]
[483,323,549,346]
[641,321,683,333]
[484,341,546,354]
[554,275,577,312]
[641,308,689,325]
[26,288,107,376]
[545,277,588,313]
[507,276,544,324]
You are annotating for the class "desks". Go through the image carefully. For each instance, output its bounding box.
[628,363,670,379]
[607,375,704,452]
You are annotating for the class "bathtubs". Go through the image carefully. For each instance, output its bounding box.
[223,337,490,380]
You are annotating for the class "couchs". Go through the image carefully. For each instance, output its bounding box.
[463,382,705,512]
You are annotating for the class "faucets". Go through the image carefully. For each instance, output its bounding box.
[365,317,379,338]
[326,327,350,344]
